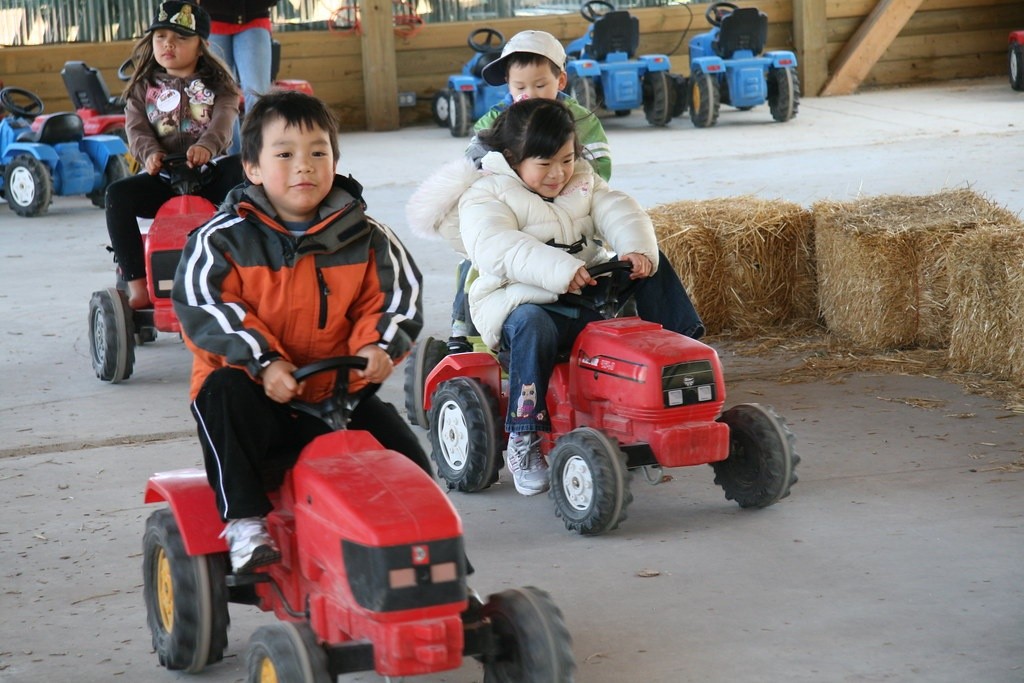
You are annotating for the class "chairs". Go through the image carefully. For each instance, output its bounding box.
[580,12,641,60]
[711,8,769,54]
[17,111,84,143]
[272,42,282,83]
[60,61,126,114]
[474,52,500,77]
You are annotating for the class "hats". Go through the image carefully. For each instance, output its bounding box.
[482,30,567,87]
[145,0,211,39]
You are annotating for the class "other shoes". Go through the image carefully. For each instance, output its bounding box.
[445,335,474,358]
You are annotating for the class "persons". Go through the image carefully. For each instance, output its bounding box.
[103,0,250,308]
[406,98,706,496]
[172,92,482,600]
[201,0,273,155]
[448,30,613,352]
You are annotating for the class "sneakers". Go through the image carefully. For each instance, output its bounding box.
[219,517,282,575]
[462,577,491,630]
[506,432,550,497]
